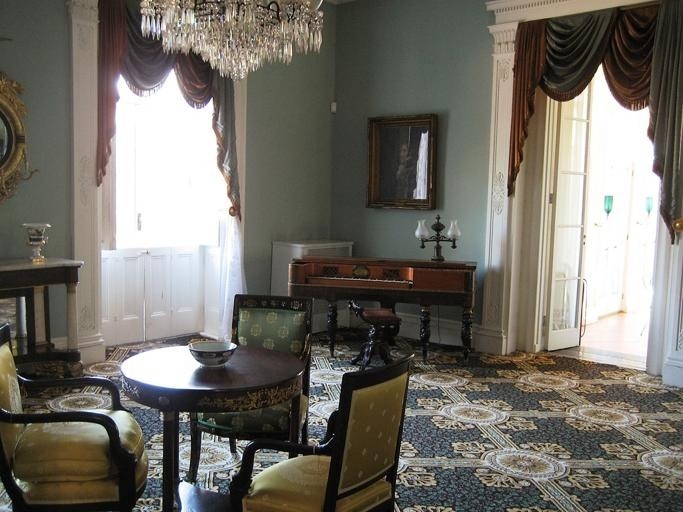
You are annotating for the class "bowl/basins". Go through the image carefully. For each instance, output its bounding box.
[187,340,238,368]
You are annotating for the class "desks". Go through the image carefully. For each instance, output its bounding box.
[0,256,84,363]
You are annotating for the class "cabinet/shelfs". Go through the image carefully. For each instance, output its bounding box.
[270,240,354,334]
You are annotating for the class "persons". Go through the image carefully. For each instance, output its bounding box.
[392,141,418,199]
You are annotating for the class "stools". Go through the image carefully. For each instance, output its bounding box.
[349,300,402,372]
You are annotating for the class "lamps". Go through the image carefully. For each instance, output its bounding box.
[140,1,324,81]
[414,215,461,264]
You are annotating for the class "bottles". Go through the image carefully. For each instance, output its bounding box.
[645,196,654,217]
[603,195,614,218]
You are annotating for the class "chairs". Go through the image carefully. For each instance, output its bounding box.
[228,355,412,511]
[230,293,314,453]
[1,324,149,511]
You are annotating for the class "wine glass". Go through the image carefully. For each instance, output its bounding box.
[22,222,52,264]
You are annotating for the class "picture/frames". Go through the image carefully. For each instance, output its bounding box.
[366,113,440,212]
[0,72,38,205]
